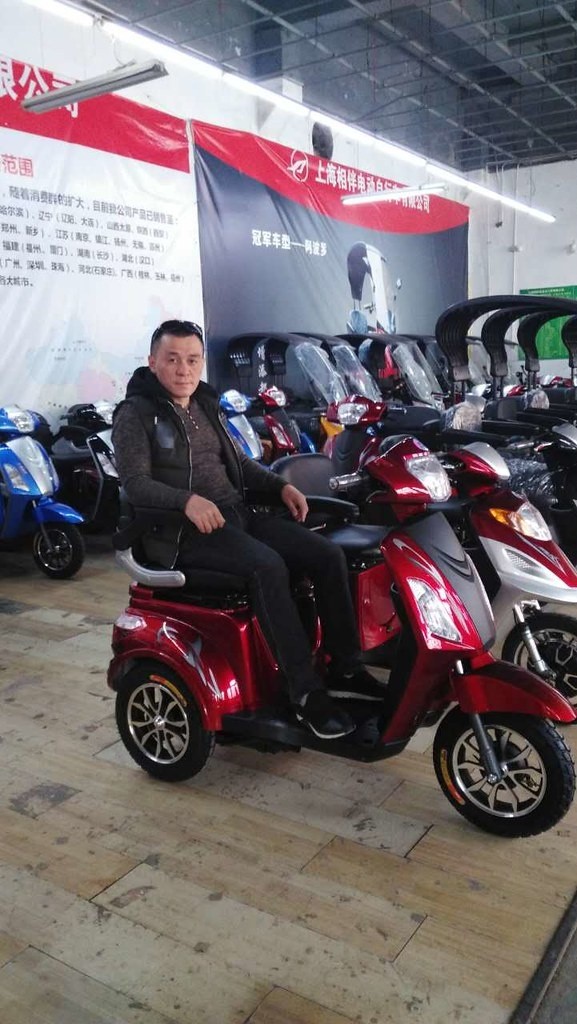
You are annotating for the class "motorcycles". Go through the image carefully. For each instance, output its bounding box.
[1,294,576,840]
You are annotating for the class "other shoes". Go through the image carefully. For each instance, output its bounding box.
[325,663,386,701]
[293,690,357,739]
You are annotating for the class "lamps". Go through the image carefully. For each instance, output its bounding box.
[340,181,447,218]
[20,55,170,116]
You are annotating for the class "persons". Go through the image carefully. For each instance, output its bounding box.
[110,319,388,740]
